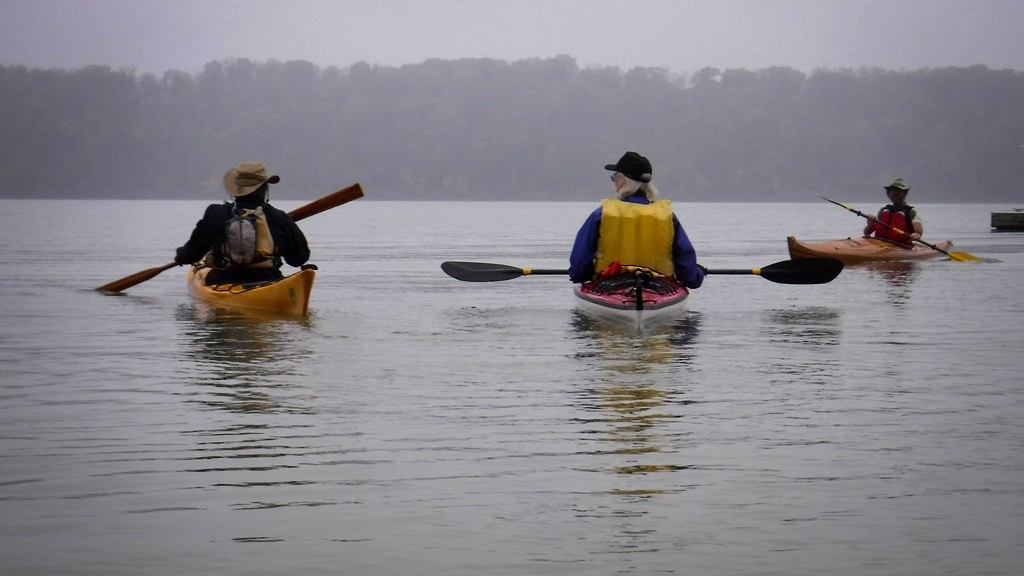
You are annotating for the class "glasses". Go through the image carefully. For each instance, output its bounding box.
[609,172,626,182]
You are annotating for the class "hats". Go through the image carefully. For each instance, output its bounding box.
[224,160,280,196]
[884,178,910,191]
[605,151,652,183]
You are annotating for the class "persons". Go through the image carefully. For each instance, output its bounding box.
[567,151,704,289]
[862,178,923,246]
[175,161,310,290]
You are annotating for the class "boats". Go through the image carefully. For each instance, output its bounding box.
[786,234,953,266]
[571,265,693,328]
[187,254,317,318]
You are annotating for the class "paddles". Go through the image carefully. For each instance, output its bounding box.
[96,180,366,295]
[813,190,978,264]
[440,254,846,287]
